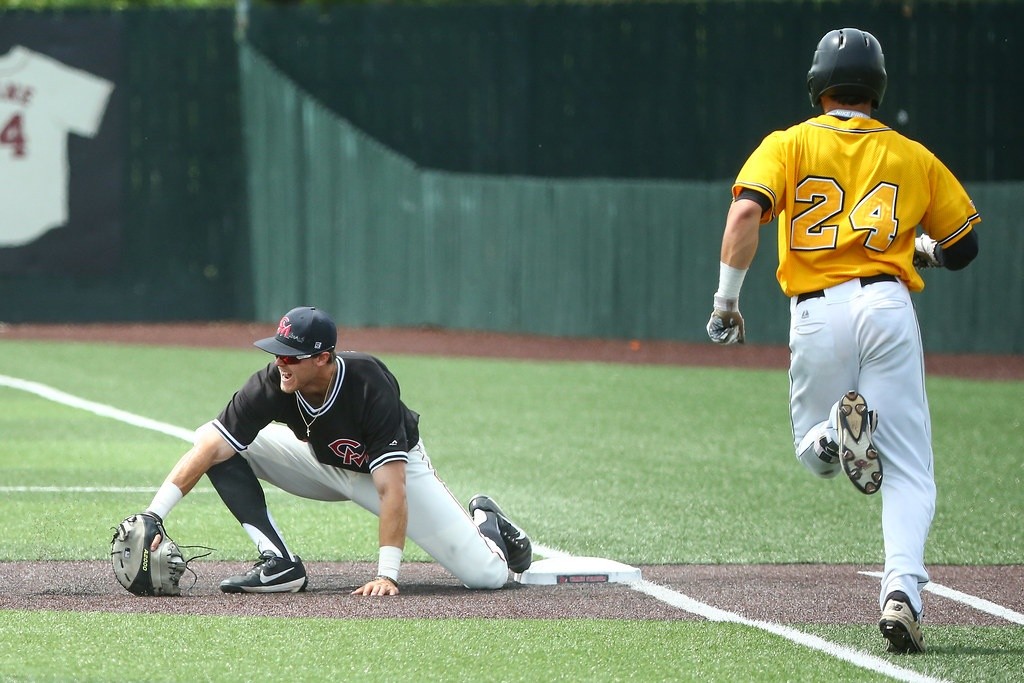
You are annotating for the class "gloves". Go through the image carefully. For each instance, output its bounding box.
[913,233,942,270]
[706,296,746,346]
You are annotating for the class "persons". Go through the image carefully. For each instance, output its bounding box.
[111,307,531,598]
[705,28,981,654]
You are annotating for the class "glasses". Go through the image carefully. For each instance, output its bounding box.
[275,345,334,365]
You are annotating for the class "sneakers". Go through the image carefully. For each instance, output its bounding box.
[468,495,533,575]
[877,590,927,654]
[218,539,309,594]
[836,390,884,495]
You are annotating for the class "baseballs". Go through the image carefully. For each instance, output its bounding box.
[171,556,183,566]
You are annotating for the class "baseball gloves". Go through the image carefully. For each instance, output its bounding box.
[111,511,186,597]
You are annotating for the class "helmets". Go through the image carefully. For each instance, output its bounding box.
[806,27,888,110]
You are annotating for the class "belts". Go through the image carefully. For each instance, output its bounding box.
[796,271,898,306]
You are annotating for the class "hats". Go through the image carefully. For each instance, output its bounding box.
[254,306,337,356]
